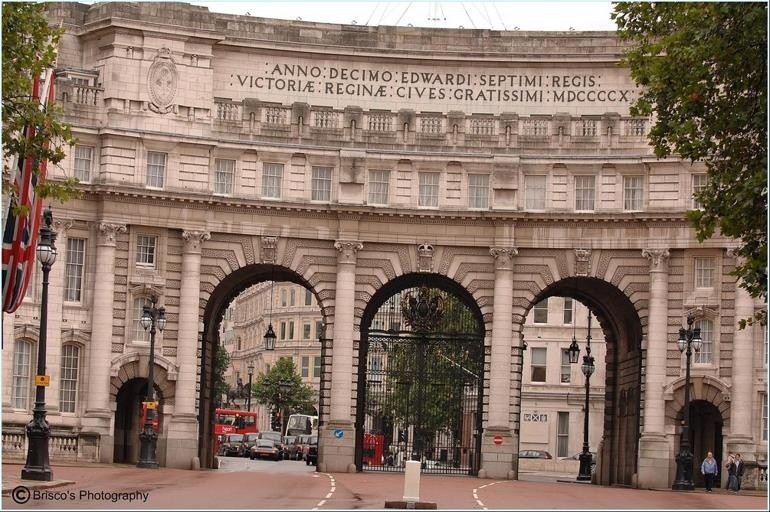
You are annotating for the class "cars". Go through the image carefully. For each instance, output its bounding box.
[561,451,597,460]
[219,431,317,466]
[519,450,552,459]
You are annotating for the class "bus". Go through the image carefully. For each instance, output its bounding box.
[212,409,259,456]
[285,415,319,436]
[362,434,385,466]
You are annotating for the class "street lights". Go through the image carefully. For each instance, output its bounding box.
[21,203,59,482]
[247,361,255,412]
[135,294,167,469]
[672,311,703,490]
[577,310,595,480]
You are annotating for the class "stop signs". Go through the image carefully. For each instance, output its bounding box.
[493,436,503,444]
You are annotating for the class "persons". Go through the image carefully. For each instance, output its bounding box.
[735,453,744,490]
[384,436,462,468]
[724,450,737,493]
[701,451,718,493]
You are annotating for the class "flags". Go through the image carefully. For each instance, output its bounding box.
[2,55,55,314]
